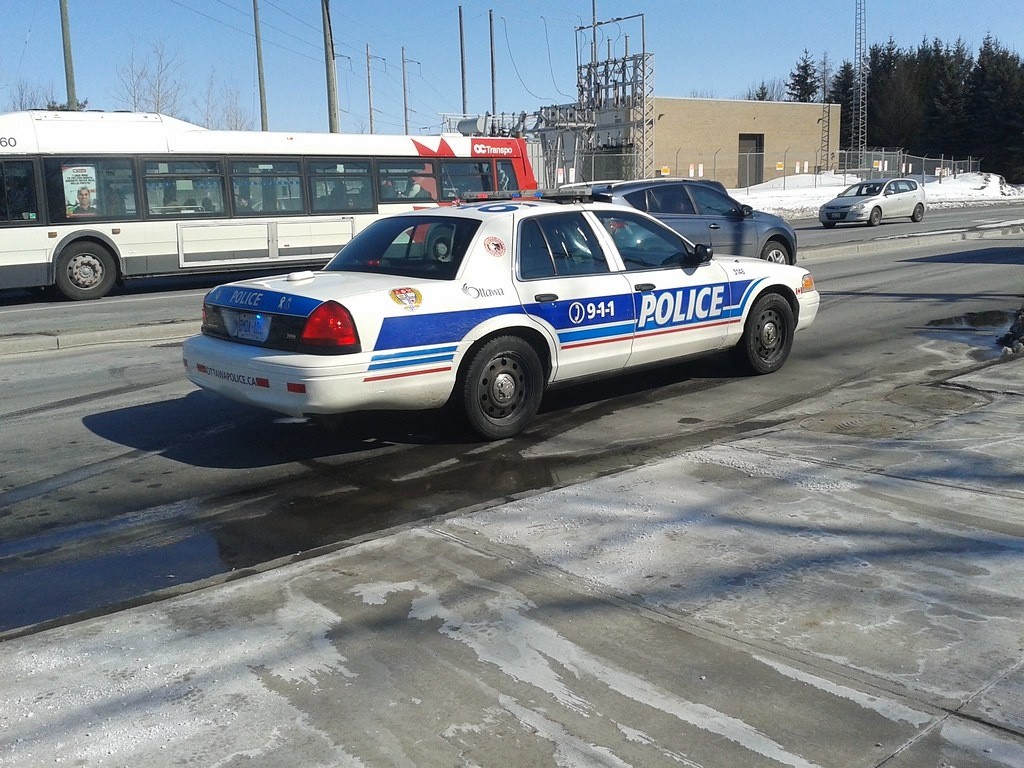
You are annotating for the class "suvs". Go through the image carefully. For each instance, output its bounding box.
[548,176,799,268]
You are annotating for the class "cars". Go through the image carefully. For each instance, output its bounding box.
[181,171,824,443]
[817,176,928,227]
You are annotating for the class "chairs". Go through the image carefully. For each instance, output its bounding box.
[866,188,874,194]
[184,187,361,212]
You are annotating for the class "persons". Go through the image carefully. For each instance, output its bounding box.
[397,172,419,198]
[183,199,200,211]
[201,198,212,211]
[6,176,33,217]
[66,187,96,215]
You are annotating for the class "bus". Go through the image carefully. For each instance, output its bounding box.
[0,108,542,304]
